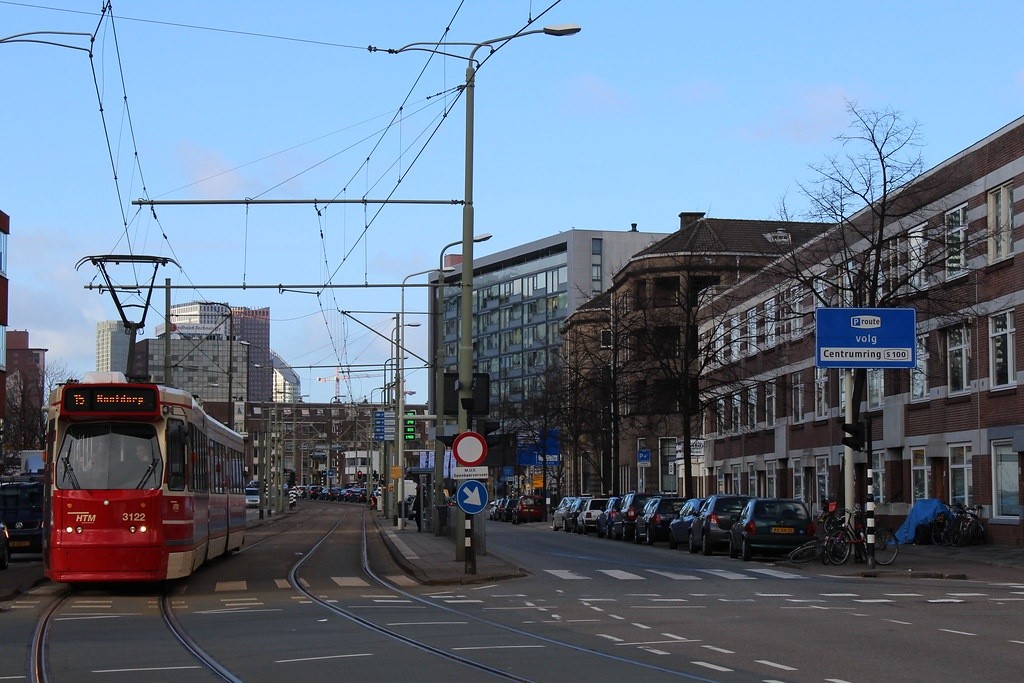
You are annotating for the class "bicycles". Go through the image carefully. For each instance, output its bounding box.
[813,495,899,566]
[929,503,984,547]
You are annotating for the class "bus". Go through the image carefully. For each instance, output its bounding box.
[43,371,247,582]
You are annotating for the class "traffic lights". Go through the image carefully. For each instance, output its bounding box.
[476,420,506,449]
[358,471,362,479]
[842,421,865,451]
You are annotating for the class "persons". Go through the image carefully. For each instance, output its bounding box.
[128,443,151,479]
[412,485,427,532]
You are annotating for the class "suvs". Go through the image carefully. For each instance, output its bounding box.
[634,496,687,546]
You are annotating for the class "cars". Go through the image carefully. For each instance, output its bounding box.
[729,499,815,561]
[489,495,546,525]
[688,494,767,555]
[668,498,707,550]
[0,469,45,570]
[246,480,288,509]
[293,486,382,503]
[552,491,667,542]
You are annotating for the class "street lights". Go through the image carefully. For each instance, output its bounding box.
[456,23,582,562]
[434,233,492,537]
[369,322,421,526]
[276,389,295,471]
[240,340,264,519]
[254,365,279,515]
[198,301,233,430]
[397,267,456,531]
